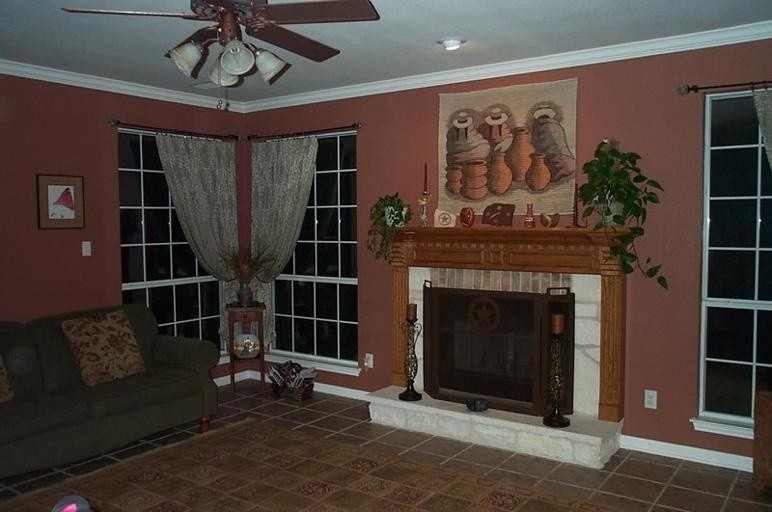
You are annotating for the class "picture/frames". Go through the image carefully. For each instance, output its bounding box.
[37,175,85,230]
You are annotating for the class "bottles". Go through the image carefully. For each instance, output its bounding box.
[524,203,534,228]
[445,126,552,201]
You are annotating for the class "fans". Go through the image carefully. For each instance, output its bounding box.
[61,0,380,63]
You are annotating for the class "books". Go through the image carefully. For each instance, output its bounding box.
[268,365,314,388]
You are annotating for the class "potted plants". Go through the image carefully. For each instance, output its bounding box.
[365,193,410,257]
[216,244,277,306]
[578,142,672,288]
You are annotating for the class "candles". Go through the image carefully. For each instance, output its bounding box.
[552,314,565,334]
[422,161,431,195]
[407,303,417,319]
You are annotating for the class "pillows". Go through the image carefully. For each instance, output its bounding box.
[0,355,15,403]
[62,310,146,386]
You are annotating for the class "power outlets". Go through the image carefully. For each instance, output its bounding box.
[82,241,92,258]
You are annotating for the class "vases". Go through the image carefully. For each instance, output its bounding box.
[445,103,575,200]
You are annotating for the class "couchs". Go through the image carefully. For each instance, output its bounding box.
[0,304,221,475]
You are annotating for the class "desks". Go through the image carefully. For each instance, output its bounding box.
[225,303,266,392]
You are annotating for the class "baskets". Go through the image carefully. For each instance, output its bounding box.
[270,363,313,401]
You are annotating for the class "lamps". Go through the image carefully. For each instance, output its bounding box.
[169,37,287,87]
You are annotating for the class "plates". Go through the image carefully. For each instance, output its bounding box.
[233,334,260,359]
